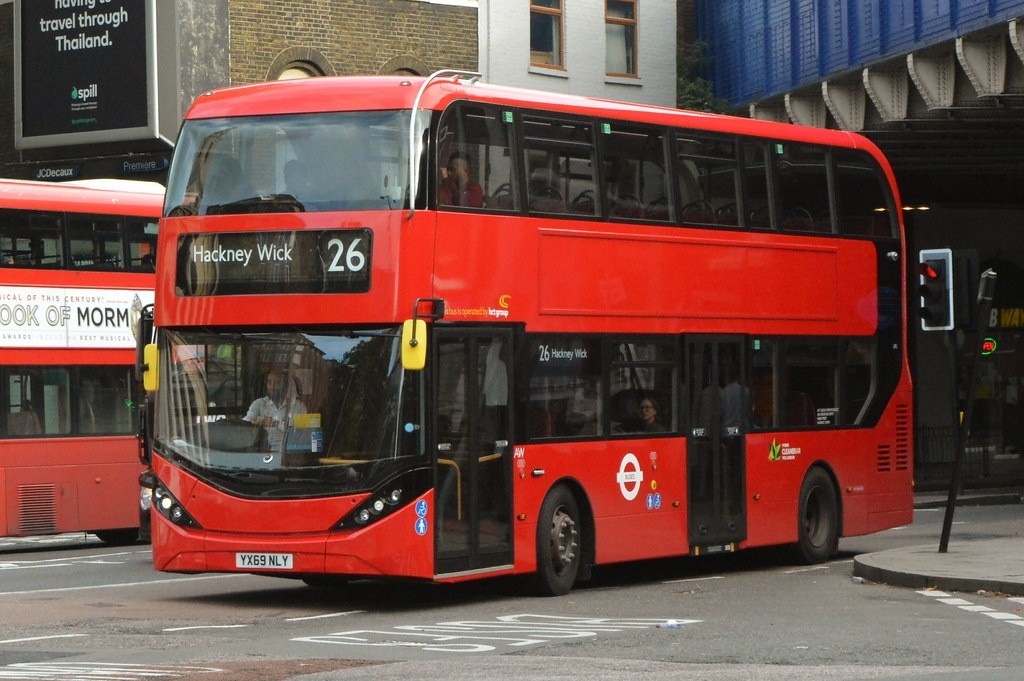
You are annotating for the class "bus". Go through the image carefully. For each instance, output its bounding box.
[136,67,916,596]
[1,175,167,548]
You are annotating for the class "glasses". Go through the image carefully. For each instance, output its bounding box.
[638,405,655,411]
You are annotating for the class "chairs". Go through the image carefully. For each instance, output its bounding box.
[483,182,812,232]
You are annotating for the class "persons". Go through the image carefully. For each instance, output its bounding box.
[439,152,485,209]
[638,397,666,433]
[563,370,622,435]
[206,153,317,208]
[242,370,308,455]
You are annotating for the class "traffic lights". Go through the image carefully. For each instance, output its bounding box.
[916,248,954,330]
